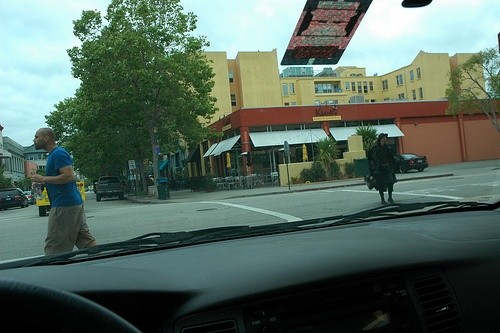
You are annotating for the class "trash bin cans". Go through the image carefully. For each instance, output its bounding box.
[156,178,172,200]
[353,157,370,178]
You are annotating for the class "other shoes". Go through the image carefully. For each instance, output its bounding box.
[381,200,388,206]
[388,197,394,203]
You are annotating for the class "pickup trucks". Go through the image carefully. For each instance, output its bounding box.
[94,175,124,202]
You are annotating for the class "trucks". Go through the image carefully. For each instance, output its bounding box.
[35,180,86,217]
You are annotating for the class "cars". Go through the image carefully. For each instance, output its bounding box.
[24,190,36,205]
[0,187,29,211]
[394,152,429,174]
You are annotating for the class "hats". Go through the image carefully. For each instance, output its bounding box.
[377,133,388,142]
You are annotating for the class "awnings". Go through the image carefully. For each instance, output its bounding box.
[249,127,330,147]
[202,134,241,156]
[160,158,168,169]
[329,123,405,140]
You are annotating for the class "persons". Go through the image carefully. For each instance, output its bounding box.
[366,132,398,203]
[30,126,97,255]
